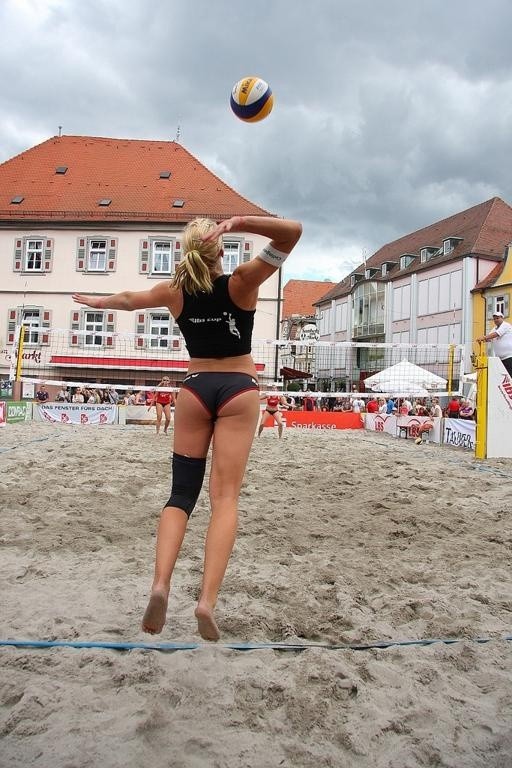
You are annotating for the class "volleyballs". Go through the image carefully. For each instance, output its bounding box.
[231,75,273,122]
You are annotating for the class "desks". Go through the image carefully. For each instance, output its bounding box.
[397,424,410,439]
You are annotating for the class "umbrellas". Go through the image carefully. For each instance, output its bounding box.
[373,381,428,413]
[363,359,449,401]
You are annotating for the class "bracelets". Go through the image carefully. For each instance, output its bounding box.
[236,217,245,230]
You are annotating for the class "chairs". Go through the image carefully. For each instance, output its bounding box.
[414,428,430,446]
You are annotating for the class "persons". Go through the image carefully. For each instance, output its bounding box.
[36,385,50,402]
[147,376,176,436]
[279,388,477,444]
[478,313,512,377]
[71,215,303,641]
[56,381,183,407]
[258,385,296,439]
